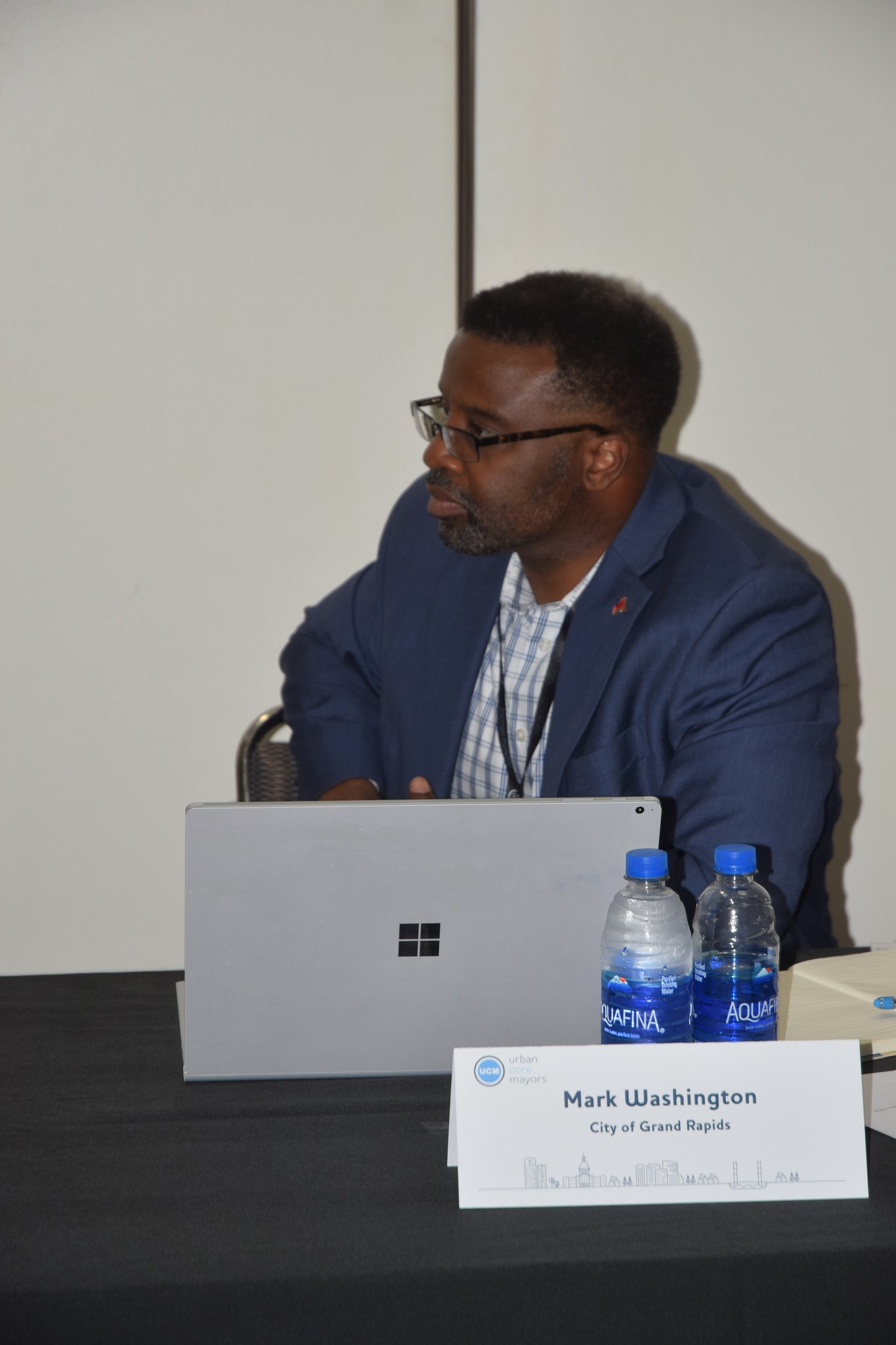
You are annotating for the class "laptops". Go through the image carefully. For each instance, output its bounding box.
[176,796,663,1081]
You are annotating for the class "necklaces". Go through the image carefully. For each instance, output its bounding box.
[497,602,574,799]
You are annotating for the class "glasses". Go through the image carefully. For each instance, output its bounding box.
[410,396,610,463]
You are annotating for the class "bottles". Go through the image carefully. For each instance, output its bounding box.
[600,848,694,1046]
[690,842,783,1045]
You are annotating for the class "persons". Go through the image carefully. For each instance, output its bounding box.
[281,271,845,971]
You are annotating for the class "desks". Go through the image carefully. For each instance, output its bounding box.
[0,973,896,1345]
